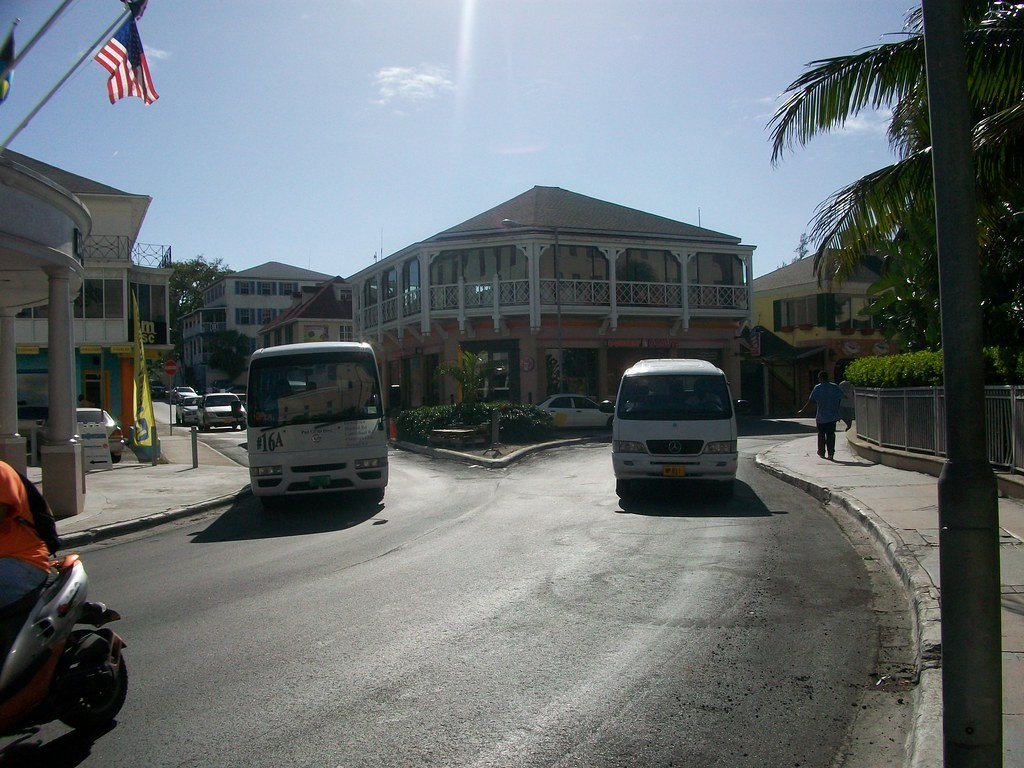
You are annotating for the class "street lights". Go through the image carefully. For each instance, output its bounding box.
[504,220,562,397]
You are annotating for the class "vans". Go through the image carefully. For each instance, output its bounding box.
[599,358,748,501]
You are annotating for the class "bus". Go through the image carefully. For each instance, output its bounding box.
[232,340,389,513]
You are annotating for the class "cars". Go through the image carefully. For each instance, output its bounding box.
[198,392,248,432]
[169,387,198,404]
[35,407,124,464]
[175,396,202,426]
[535,394,615,431]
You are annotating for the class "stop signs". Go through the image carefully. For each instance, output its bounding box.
[164,360,177,375]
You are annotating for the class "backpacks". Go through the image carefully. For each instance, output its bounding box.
[14,467,60,554]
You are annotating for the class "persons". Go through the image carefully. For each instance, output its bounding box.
[798,370,839,459]
[0,457,54,621]
[839,373,855,432]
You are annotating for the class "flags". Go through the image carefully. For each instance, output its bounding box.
[134,293,160,464]
[93,19,160,107]
[0,31,15,105]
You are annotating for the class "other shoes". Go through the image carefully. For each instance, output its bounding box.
[819,454,835,460]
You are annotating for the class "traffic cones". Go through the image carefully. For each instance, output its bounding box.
[389,417,397,439]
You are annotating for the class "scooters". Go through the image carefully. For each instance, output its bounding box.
[1,553,128,736]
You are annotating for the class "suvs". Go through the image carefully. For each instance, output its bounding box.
[149,380,166,397]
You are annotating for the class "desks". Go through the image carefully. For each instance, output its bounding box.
[432,428,476,451]
